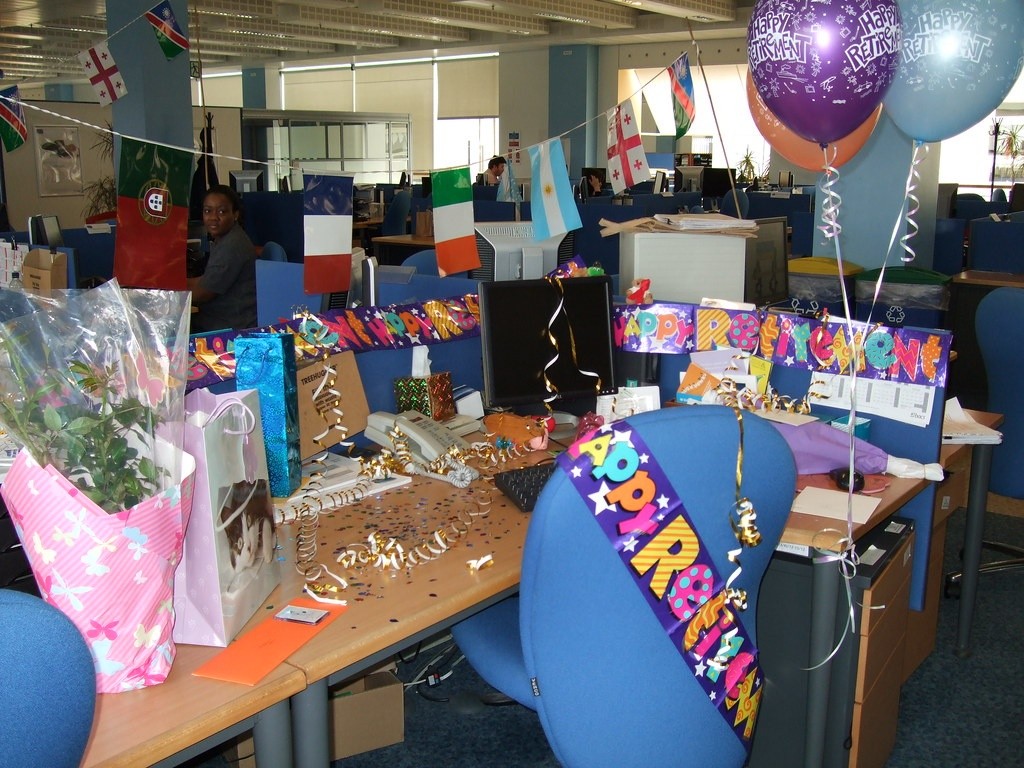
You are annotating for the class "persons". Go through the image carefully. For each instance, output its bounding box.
[187,185,256,331]
[484,155,507,184]
[584,170,605,196]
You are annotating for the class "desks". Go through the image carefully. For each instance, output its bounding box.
[372,235,434,261]
[770,412,1001,768]
[92,441,534,768]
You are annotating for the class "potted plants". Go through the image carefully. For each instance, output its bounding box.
[0,337,194,691]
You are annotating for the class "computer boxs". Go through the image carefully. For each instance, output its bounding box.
[618,229,745,307]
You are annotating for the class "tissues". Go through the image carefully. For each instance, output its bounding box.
[392,344,456,425]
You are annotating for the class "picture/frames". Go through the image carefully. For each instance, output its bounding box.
[34,124,84,198]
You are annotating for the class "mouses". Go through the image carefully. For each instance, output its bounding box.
[829,468,864,491]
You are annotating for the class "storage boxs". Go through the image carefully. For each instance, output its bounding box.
[618,228,749,304]
[22,249,67,296]
[176,390,279,645]
[235,337,303,495]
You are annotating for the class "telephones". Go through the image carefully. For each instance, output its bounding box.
[365,409,472,469]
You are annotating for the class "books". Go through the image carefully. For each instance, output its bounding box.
[676,346,773,396]
[393,371,455,423]
[942,395,1003,444]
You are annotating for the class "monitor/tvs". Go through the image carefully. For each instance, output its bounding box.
[467,221,575,282]
[581,167,606,189]
[477,275,620,416]
[318,247,380,314]
[398,172,411,187]
[650,166,736,211]
[279,175,292,192]
[935,183,958,219]
[744,216,789,307]
[229,170,263,194]
[28,215,66,249]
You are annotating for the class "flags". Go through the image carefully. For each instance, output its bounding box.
[0,85,28,152]
[113,136,195,291]
[302,168,355,295]
[605,99,651,194]
[145,0,191,63]
[78,40,128,108]
[430,166,481,278]
[528,137,583,241]
[666,52,696,141]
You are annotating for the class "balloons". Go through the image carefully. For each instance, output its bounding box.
[745,70,884,175]
[747,0,904,149]
[882,0,1024,146]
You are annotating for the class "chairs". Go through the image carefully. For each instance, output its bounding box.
[376,271,480,307]
[259,243,286,261]
[0,220,116,288]
[453,408,798,768]
[235,181,532,247]
[255,259,323,328]
[572,152,815,273]
[0,587,97,768]
[400,251,468,281]
[956,288,1024,660]
[932,184,1024,277]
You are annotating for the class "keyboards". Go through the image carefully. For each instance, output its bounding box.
[494,460,557,512]
